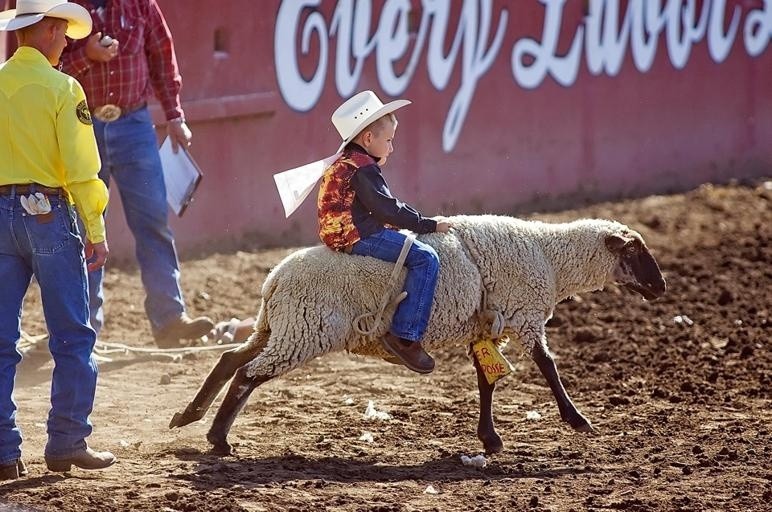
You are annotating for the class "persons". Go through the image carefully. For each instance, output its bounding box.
[50,0,214,350]
[315,90,453,374]
[0,0,117,481]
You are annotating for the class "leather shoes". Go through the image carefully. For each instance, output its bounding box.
[46,445,117,472]
[154,310,215,349]
[0,458,28,478]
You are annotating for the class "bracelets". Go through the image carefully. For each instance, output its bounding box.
[170,117,186,123]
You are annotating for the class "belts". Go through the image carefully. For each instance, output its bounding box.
[90,100,146,124]
[0,184,66,196]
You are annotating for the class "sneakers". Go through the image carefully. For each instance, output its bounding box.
[380,332,435,375]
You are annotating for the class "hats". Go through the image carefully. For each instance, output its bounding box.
[331,90,412,155]
[0,0,93,41]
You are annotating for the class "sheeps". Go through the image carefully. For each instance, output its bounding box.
[168,213,666,456]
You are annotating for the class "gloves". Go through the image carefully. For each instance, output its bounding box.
[20,193,53,216]
